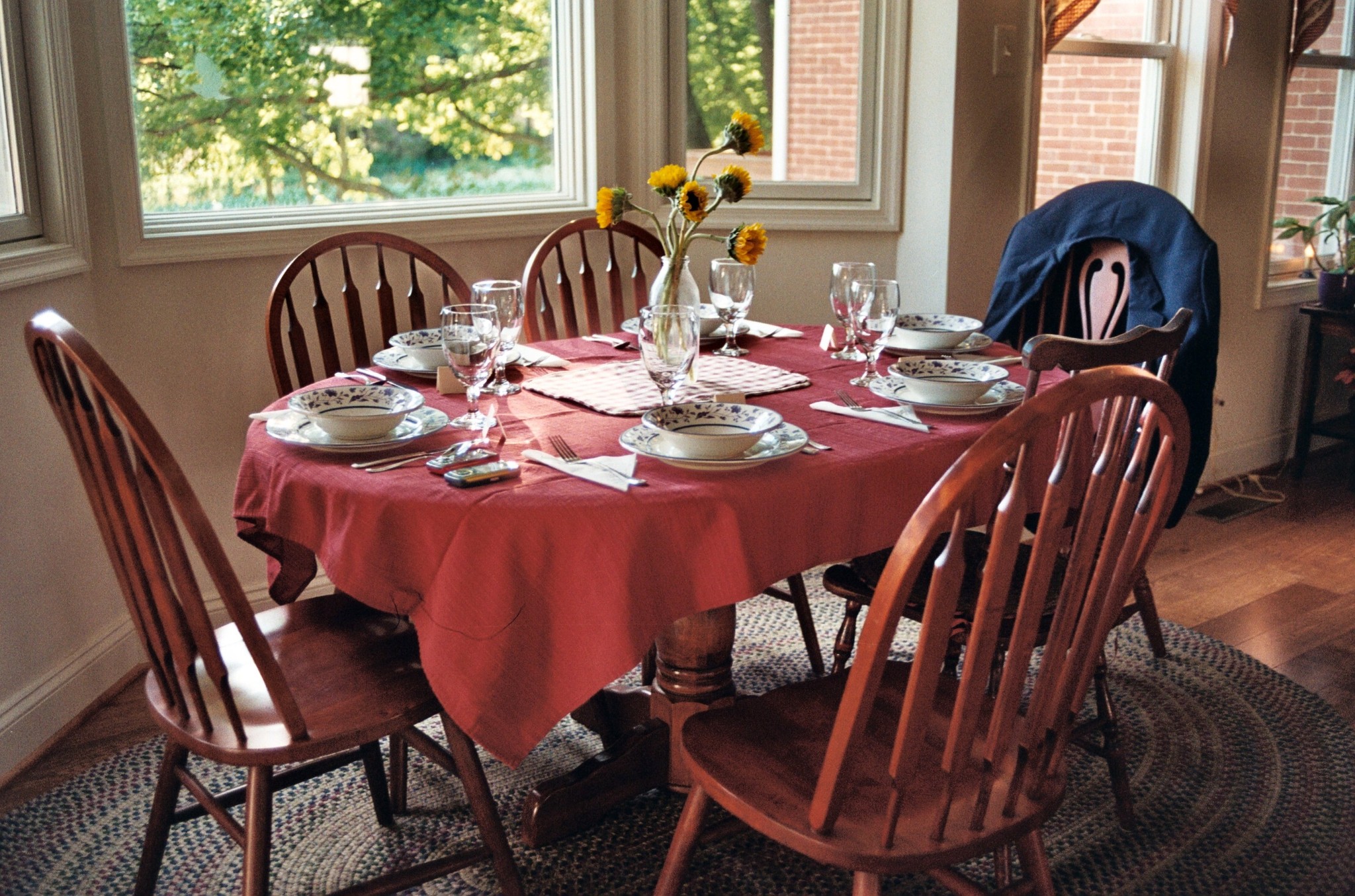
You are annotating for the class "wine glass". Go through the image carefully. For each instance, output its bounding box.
[471,279,525,396]
[829,261,900,386]
[439,303,502,431]
[638,305,701,408]
[708,257,756,356]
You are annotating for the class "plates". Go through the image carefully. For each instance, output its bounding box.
[267,412,449,454]
[372,346,521,379]
[868,375,1029,416]
[619,423,809,472]
[621,317,751,345]
[874,335,994,358]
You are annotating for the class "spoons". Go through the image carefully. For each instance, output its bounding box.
[335,372,388,386]
[582,335,631,350]
[365,442,472,473]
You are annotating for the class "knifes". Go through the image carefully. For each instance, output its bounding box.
[593,334,640,351]
[350,438,485,469]
[355,367,419,392]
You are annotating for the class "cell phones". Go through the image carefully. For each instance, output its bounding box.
[444,459,521,487]
[425,448,501,473]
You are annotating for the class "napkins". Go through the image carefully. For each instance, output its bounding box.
[512,441,645,493]
[809,388,934,441]
[716,316,808,352]
[506,342,575,375]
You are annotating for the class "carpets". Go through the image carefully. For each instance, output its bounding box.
[0,565,1355,896]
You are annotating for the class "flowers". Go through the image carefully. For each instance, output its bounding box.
[594,105,775,266]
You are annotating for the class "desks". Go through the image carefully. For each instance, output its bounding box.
[229,314,1102,860]
[1288,306,1355,482]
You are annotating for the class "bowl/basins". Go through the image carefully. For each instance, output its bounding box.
[287,386,426,439]
[641,403,784,459]
[699,302,724,337]
[893,312,983,350]
[389,325,484,369]
[887,360,1009,405]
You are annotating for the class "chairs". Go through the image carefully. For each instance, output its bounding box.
[651,363,1194,896]
[827,173,1228,684]
[817,305,1202,838]
[519,216,666,345]
[262,226,477,400]
[21,302,524,896]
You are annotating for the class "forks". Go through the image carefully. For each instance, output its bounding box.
[549,434,647,485]
[835,389,935,428]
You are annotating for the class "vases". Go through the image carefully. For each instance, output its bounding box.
[644,254,705,383]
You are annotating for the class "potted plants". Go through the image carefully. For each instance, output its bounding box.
[1272,193,1355,306]
